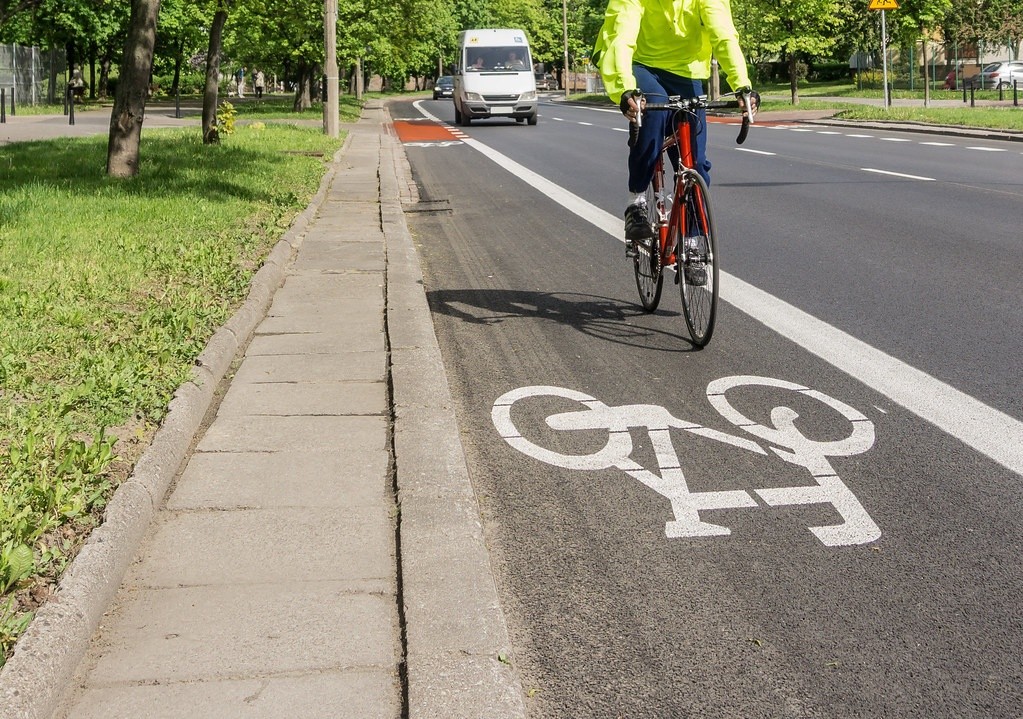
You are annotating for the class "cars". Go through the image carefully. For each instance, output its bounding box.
[534,72,559,91]
[432,76,454,100]
[975,60,1023,90]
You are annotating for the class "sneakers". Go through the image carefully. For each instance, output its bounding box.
[674,247,707,287]
[624,201,652,239]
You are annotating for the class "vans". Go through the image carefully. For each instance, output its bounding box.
[454,29,544,126]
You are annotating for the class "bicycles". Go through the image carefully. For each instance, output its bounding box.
[624,84,755,348]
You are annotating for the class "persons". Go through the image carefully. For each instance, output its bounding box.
[505,51,523,69]
[234,65,265,98]
[472,57,486,70]
[589,0,759,286]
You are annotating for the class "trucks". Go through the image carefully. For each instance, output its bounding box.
[945,64,990,90]
